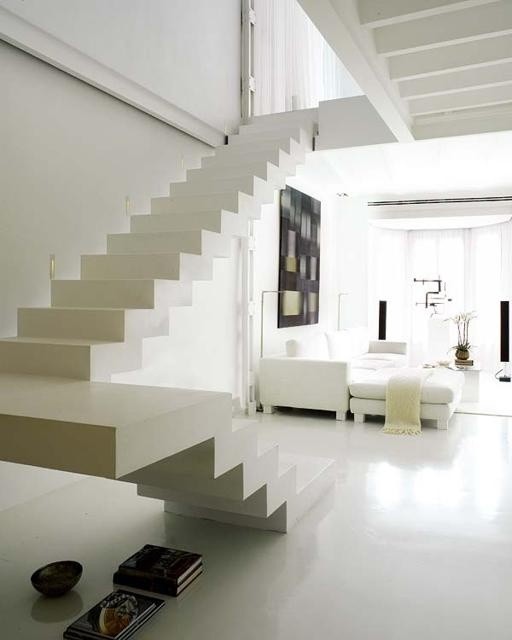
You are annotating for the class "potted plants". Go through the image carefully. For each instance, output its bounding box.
[448,342,472,360]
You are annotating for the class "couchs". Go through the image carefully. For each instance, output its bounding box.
[258,339,410,422]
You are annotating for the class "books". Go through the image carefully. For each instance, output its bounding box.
[119,544,203,586]
[113,563,204,597]
[63,588,166,640]
[68,591,156,640]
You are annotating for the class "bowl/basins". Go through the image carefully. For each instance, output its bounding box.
[31,560,83,596]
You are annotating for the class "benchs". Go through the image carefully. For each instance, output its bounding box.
[350,366,466,430]
